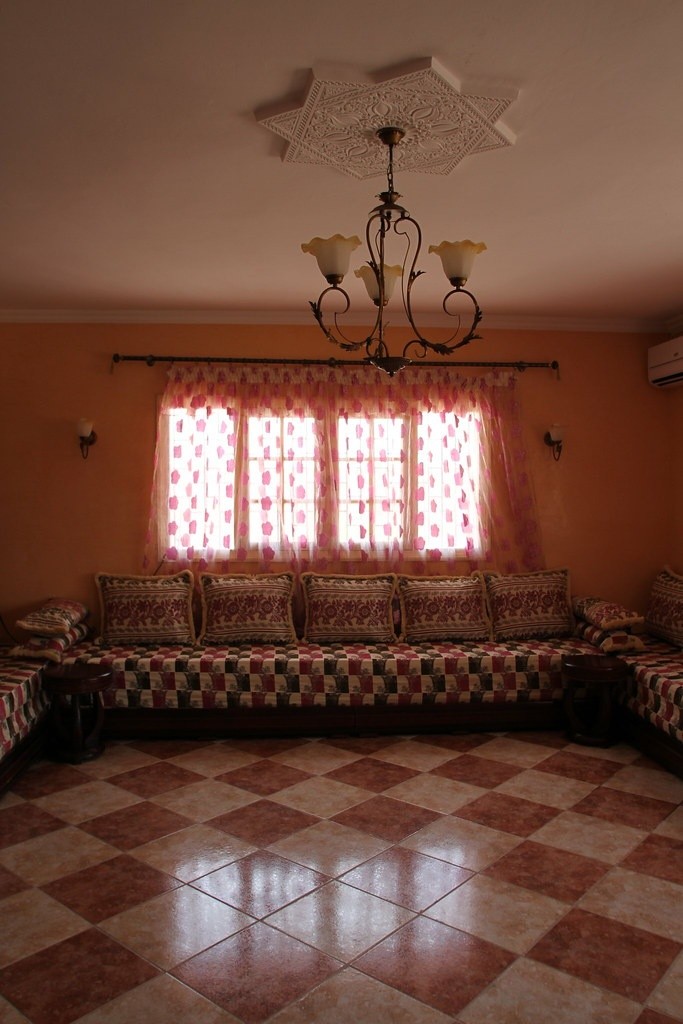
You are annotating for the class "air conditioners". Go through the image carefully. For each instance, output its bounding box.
[646,335,683,390]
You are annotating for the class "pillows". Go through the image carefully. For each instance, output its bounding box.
[646,564,683,648]
[94,570,198,647]
[468,568,576,640]
[15,595,91,637]
[196,570,301,645]
[8,622,91,666]
[568,616,650,657]
[298,571,400,643]
[392,569,495,645]
[569,589,646,633]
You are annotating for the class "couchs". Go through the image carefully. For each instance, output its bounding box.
[1,596,682,760]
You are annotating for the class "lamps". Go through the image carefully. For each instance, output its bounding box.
[543,421,565,462]
[299,126,488,379]
[76,417,98,459]
